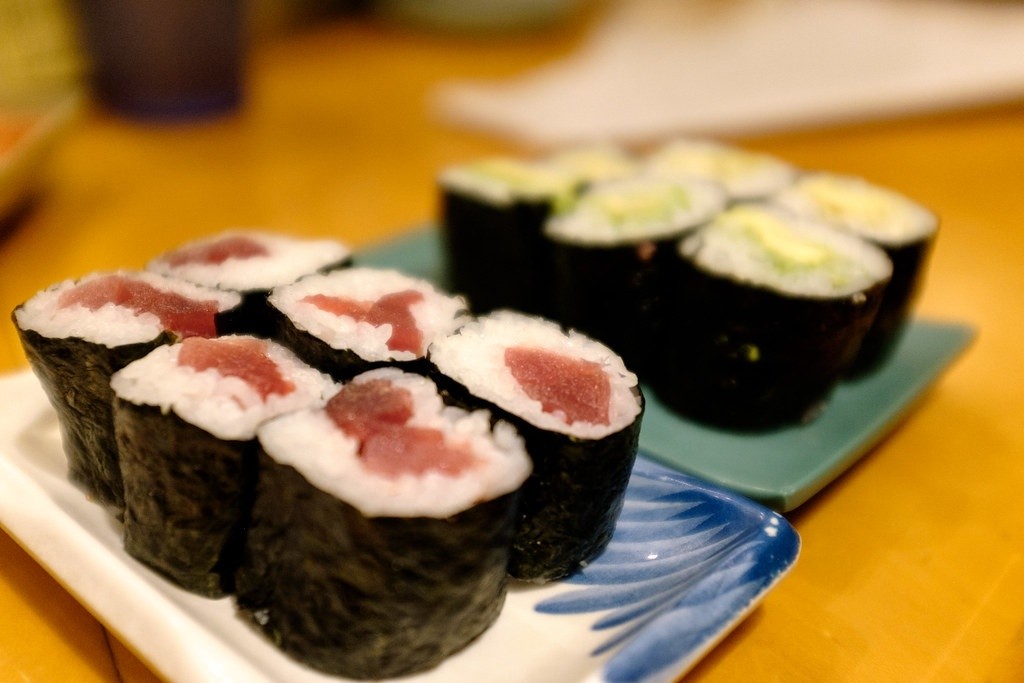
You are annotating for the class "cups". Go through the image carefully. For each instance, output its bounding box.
[72,0,241,117]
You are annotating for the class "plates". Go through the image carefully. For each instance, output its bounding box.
[352,227,978,510]
[0,366,799,683]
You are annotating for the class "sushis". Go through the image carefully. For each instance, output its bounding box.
[440,138,938,433]
[12,234,646,681]
